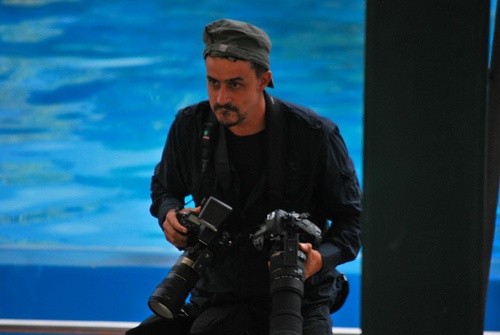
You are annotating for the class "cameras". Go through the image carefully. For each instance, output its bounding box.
[147,196,240,320]
[251,209,322,335]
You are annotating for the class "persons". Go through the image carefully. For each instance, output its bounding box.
[125,18,361,335]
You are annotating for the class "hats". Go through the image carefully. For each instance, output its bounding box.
[202,18,274,89]
